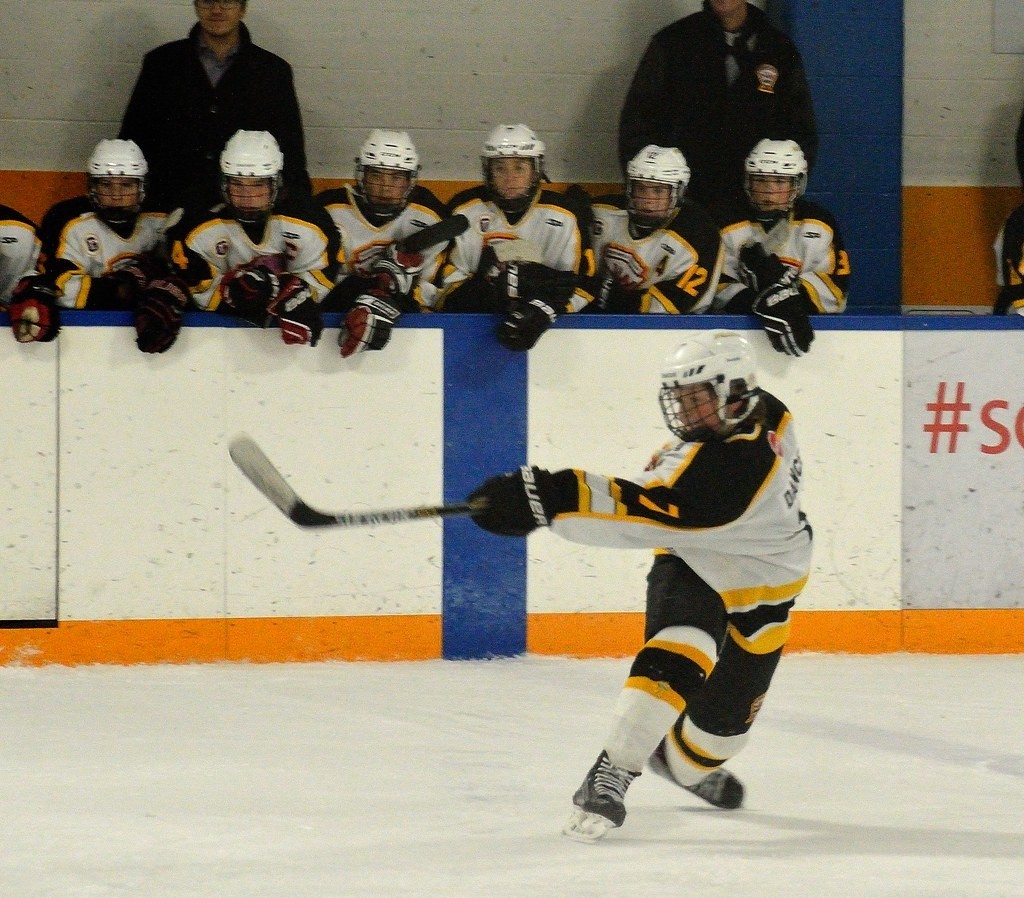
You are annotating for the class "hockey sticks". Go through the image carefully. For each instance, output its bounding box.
[231,430,488,531]
[397,217,472,251]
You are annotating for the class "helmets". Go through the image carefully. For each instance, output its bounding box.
[87,139,149,222]
[742,137,807,211]
[630,145,690,230]
[658,330,759,443]
[353,128,419,211]
[216,130,283,222]
[481,121,546,209]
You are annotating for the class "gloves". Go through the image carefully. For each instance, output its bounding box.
[338,288,400,358]
[476,246,538,302]
[587,272,650,317]
[369,239,424,295]
[136,271,191,356]
[751,284,814,358]
[467,465,560,537]
[9,274,61,343]
[497,301,560,351]
[222,265,279,314]
[734,236,800,294]
[265,276,325,348]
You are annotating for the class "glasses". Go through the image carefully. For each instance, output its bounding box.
[194,0,236,10]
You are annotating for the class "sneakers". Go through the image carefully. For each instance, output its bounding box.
[563,750,642,841]
[647,734,743,810]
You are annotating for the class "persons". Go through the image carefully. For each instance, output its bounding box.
[117,0,314,215]
[585,145,726,314]
[993,110,1024,318]
[714,138,850,356]
[39,138,187,354]
[617,0,817,226]
[0,203,61,343]
[428,124,595,350]
[163,129,340,347]
[468,331,812,845]
[317,128,452,359]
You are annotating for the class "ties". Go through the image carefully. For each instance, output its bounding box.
[724,25,753,74]
